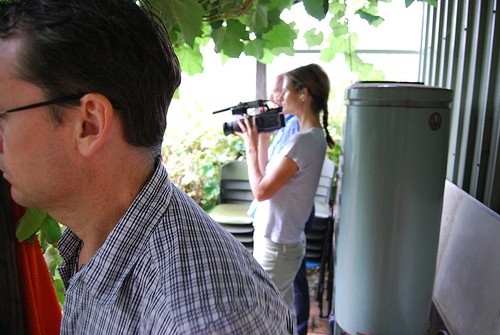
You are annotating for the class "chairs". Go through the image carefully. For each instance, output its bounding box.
[207,155,337,319]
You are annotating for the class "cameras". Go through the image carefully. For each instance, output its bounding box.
[213,100,286,135]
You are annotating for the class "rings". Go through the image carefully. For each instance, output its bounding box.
[242,128,246,132]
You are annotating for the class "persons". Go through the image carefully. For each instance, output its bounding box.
[234,64,336,335]
[0,0,297,335]
[247,73,310,335]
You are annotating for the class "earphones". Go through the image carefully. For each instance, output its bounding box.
[299,93,305,100]
